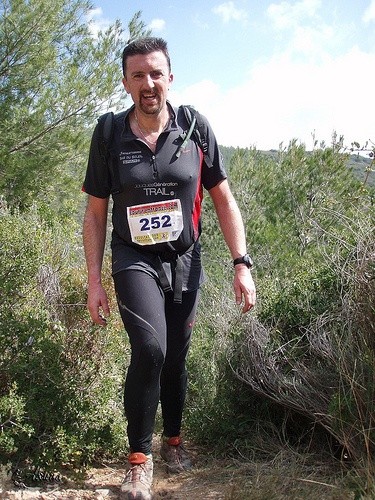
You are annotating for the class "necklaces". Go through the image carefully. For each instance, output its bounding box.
[134,110,156,145]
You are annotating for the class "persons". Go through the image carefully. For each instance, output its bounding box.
[80,36,257,500]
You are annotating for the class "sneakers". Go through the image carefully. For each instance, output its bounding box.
[119,452,153,500]
[159,432,186,473]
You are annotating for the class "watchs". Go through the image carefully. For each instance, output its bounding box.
[233,253,253,269]
